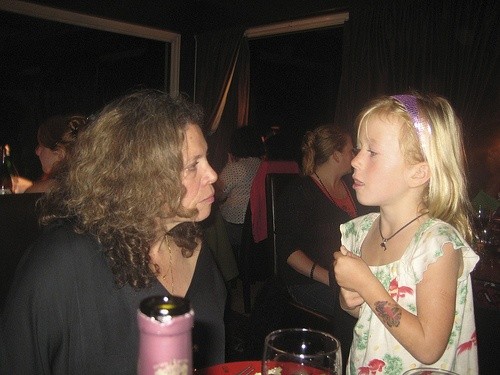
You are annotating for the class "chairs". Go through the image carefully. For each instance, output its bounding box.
[233,159,361,367]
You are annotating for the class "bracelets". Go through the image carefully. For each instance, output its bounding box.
[310,262,317,280]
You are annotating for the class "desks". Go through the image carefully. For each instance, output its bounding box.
[471,214,500,314]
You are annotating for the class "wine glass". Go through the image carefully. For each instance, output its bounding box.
[472,204,493,261]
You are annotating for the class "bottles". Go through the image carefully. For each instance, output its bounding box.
[138,293,194,375]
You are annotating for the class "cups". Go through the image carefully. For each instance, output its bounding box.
[261,328,342,375]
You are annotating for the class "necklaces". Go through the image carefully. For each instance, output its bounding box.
[164,235,177,295]
[313,170,357,216]
[379,211,430,251]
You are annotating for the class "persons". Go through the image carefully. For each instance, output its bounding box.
[275,123,381,317]
[24,114,84,194]
[0,89,228,375]
[216,128,262,243]
[331,93,480,375]
